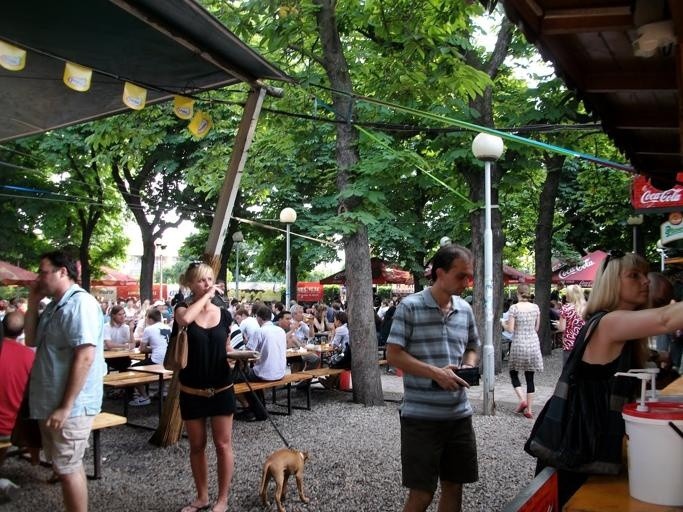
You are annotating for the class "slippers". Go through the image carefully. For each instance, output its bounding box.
[179,500,210,512]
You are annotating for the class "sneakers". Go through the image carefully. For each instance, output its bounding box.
[106,390,155,406]
[515,400,532,418]
[319,377,333,389]
[0,479,21,501]
[235,411,257,421]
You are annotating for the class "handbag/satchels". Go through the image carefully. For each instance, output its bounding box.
[523,366,635,479]
[328,342,351,369]
[11,376,42,448]
[164,328,189,370]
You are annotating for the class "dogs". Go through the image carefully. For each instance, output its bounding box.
[259,447,310,512]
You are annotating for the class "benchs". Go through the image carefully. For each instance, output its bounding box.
[0,346,387,479]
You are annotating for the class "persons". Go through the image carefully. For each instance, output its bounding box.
[214,280,404,422]
[465,249,683,511]
[1,296,54,437]
[25,251,104,511]
[171,263,260,511]
[386,244,482,511]
[94,288,194,406]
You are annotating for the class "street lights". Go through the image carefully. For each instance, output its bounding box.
[626,212,644,253]
[471,131,504,416]
[156,241,167,301]
[440,235,452,246]
[657,239,667,272]
[233,231,243,300]
[280,207,297,312]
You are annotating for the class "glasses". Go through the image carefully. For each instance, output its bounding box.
[602,249,626,272]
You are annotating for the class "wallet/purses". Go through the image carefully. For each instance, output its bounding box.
[431,367,481,389]
